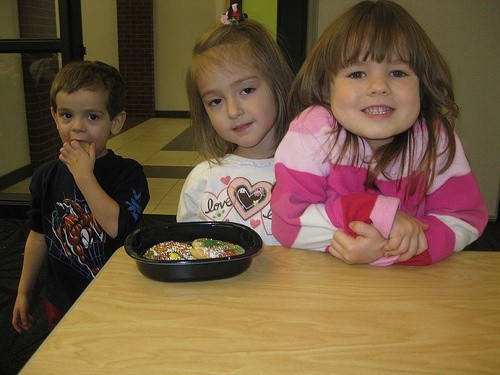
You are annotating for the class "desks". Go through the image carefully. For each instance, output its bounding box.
[16,239,500,375]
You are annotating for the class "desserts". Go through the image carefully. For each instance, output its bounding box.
[143,240,194,260]
[192,237,246,258]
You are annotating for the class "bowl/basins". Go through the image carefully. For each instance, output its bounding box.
[124,221,263,282]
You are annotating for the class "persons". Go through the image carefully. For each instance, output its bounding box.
[175,4,311,247]
[11,60,149,333]
[270,0,488,266]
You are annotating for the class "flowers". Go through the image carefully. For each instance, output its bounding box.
[219,3,247,27]
